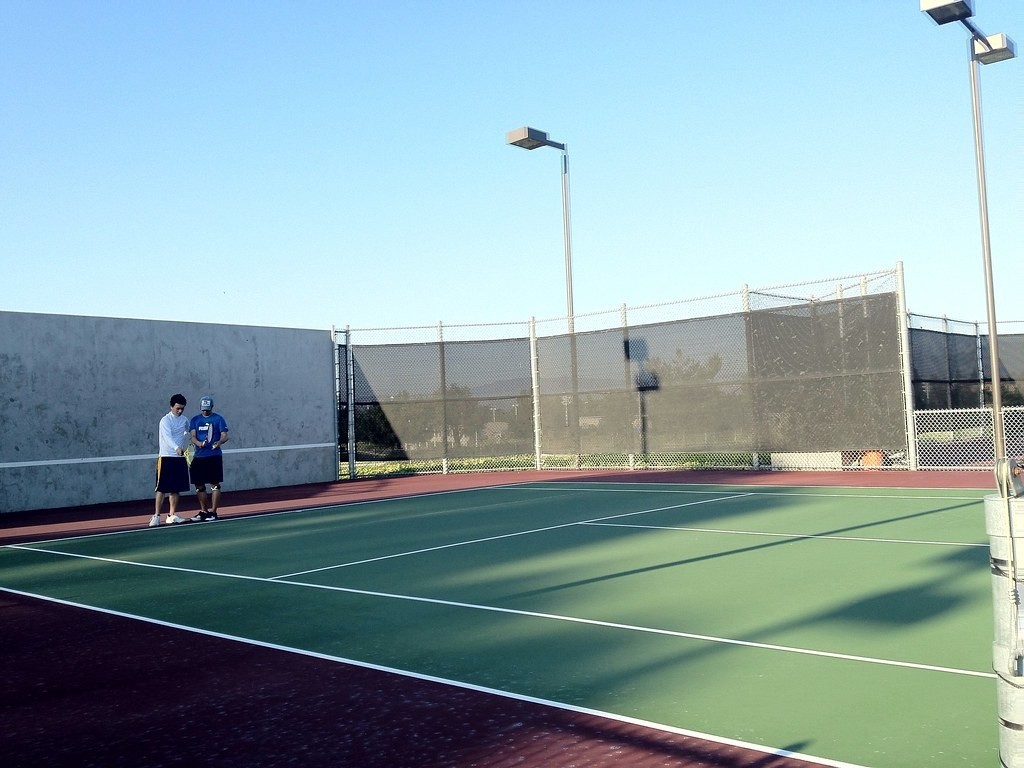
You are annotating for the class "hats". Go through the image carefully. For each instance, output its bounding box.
[199,396,214,411]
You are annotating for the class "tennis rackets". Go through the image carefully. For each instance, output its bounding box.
[202,422,214,449]
[182,443,196,467]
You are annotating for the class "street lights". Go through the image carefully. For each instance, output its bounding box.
[507,126,581,468]
[921,0,1024,768]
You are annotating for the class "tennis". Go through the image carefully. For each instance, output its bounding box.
[183,430,189,436]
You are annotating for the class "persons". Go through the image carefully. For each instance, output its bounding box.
[148,394,190,527]
[189,396,228,522]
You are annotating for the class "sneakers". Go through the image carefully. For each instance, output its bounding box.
[190,510,208,521]
[166,514,187,524]
[204,512,218,521]
[149,514,162,526]
